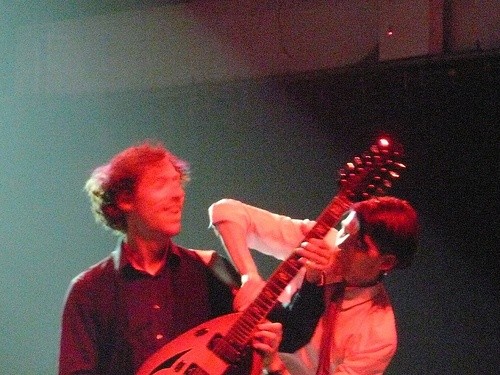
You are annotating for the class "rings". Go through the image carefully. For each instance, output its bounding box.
[304,260,316,269]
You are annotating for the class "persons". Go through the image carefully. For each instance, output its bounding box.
[58,142,334,375]
[207,190,421,374]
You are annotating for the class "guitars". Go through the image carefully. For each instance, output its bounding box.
[152,134,405,375]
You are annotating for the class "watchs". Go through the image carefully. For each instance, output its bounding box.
[268,361,286,375]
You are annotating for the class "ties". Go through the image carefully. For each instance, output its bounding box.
[317,280,345,373]
